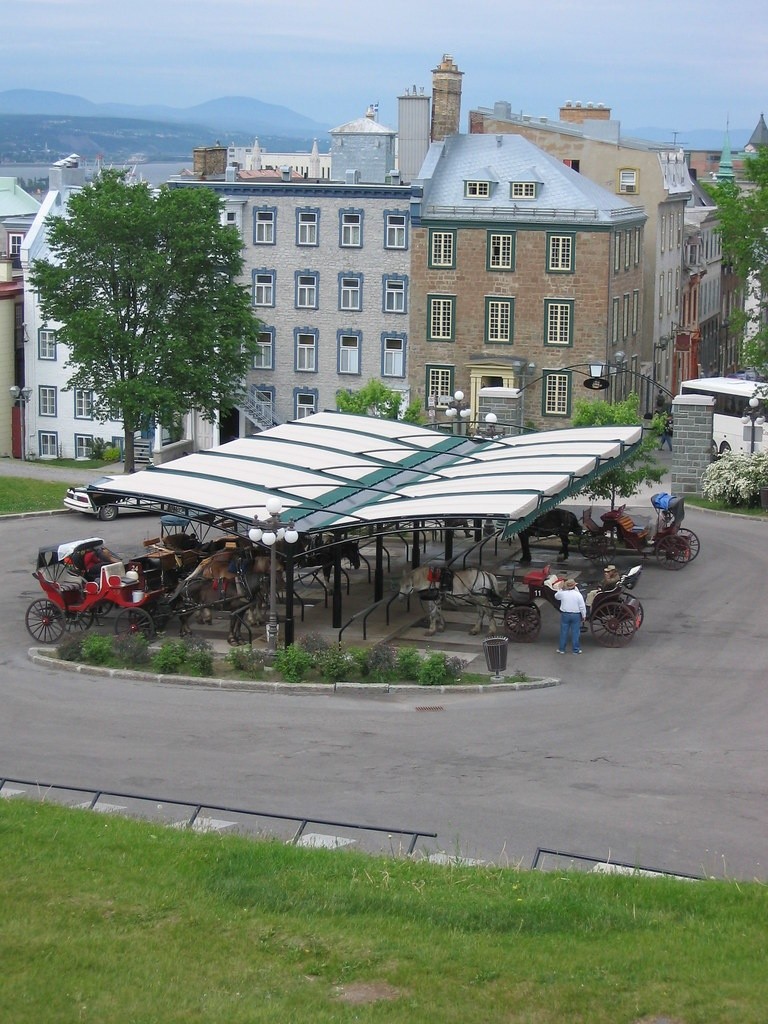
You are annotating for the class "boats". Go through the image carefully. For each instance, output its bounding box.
[126,153,147,165]
[53,153,80,168]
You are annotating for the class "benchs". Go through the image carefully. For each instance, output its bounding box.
[38,563,83,605]
[103,561,141,602]
[616,514,650,540]
[652,517,682,541]
[81,561,122,607]
[591,565,643,620]
[161,533,199,553]
[128,556,162,578]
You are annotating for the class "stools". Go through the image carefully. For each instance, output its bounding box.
[522,564,552,587]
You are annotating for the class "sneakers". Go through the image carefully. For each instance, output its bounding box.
[572,650,582,654]
[556,649,564,654]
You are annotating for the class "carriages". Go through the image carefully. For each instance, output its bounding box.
[515,492,700,570]
[127,515,360,628]
[25,536,287,647]
[397,563,644,648]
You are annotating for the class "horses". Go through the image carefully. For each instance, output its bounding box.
[168,530,360,649]
[517,508,583,566]
[398,566,503,638]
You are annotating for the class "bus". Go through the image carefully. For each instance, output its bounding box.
[679,376,768,462]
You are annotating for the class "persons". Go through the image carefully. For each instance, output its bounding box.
[586,566,620,617]
[656,390,665,413]
[658,413,673,451]
[555,579,586,654]
[73,549,101,582]
[657,510,672,533]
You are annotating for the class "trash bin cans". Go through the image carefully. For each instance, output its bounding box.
[483,635,508,683]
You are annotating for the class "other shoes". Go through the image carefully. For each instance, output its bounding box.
[670,449,672,451]
[658,449,663,451]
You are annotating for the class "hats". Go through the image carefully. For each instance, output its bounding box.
[604,565,616,571]
[563,579,578,589]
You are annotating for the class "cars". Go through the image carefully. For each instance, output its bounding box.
[64,476,158,521]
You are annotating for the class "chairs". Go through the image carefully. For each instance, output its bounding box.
[600,504,626,522]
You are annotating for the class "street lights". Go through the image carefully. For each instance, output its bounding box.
[741,398,765,456]
[446,390,472,435]
[9,386,33,461]
[512,361,537,434]
[249,497,298,672]
